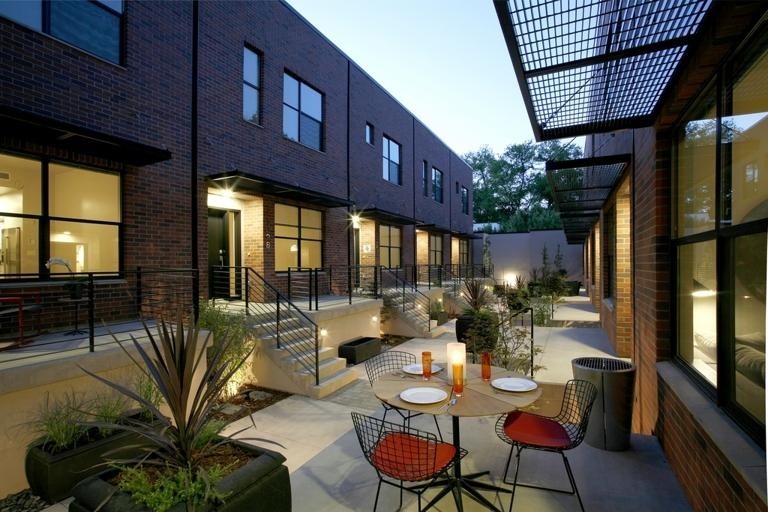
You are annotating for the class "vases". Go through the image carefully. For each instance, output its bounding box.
[68,283,84,299]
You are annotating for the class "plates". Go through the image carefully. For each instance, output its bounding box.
[399,387,448,405]
[402,363,441,376]
[490,377,538,393]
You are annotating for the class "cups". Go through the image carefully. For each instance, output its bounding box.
[481,351,492,381]
[421,351,432,381]
[452,362,464,398]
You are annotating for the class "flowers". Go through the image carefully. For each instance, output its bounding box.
[46,257,86,288]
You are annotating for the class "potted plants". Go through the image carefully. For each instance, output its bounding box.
[507,275,530,313]
[455,278,498,353]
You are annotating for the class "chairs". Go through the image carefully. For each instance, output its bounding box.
[351,350,599,511]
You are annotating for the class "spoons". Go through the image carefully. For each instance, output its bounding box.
[445,399,457,414]
